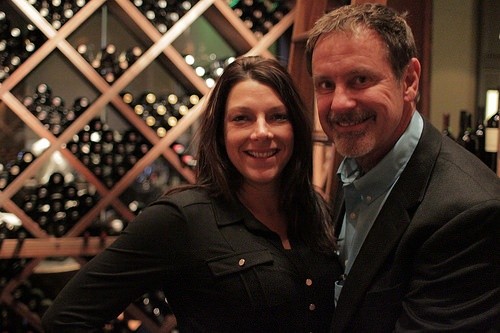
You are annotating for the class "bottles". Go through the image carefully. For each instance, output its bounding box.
[458,89,499,173]
[0,0,292,333]
[441,112,457,143]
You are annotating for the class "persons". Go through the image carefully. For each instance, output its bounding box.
[303,0,500,331]
[44,55,332,332]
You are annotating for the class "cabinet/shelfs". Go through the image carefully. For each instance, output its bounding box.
[0,1,329,333]
